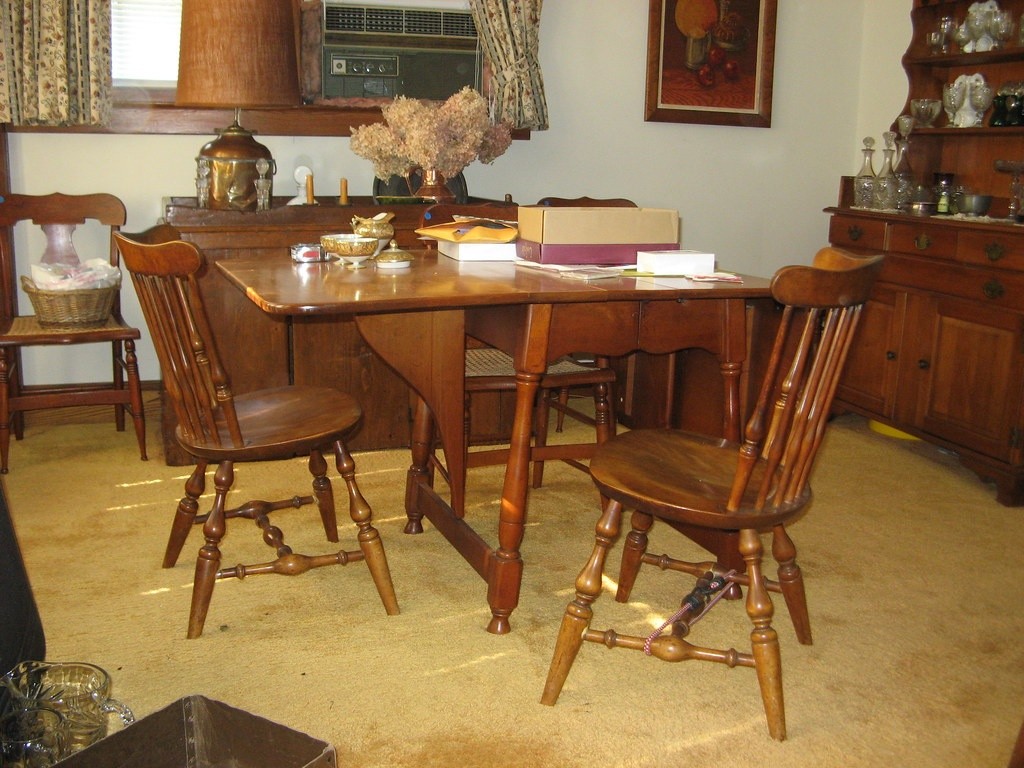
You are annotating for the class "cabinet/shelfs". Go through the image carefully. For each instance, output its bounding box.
[823,0,1024,507]
[157,205,516,467]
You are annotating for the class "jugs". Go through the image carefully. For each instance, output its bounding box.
[0,661,135,752]
[354,210,395,257]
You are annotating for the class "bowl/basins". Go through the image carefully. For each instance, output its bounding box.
[334,238,379,268]
[955,193,992,217]
[319,233,362,265]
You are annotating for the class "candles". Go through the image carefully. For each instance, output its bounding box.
[306,175,314,205]
[340,178,347,205]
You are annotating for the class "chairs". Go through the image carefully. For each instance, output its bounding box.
[112,196,886,741]
[0,192,148,474]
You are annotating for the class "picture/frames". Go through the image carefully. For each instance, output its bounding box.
[644,0,777,128]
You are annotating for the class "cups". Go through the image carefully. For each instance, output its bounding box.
[0,708,71,768]
[911,97,942,129]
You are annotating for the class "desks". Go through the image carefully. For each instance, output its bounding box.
[214,249,772,635]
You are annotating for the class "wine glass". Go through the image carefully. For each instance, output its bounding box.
[942,83,966,128]
[969,81,993,128]
[926,9,1014,57]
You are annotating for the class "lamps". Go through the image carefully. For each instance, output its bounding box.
[174,0,302,211]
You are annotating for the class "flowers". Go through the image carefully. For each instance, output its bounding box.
[349,84,512,186]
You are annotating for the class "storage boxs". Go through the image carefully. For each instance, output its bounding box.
[516,205,681,266]
[636,249,715,277]
[438,240,516,261]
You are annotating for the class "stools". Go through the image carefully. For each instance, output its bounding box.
[47,694,337,768]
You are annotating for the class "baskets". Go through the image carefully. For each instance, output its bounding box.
[20,269,122,330]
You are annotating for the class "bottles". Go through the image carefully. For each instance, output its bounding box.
[852,116,954,216]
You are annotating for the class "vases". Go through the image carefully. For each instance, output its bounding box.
[405,164,456,204]
